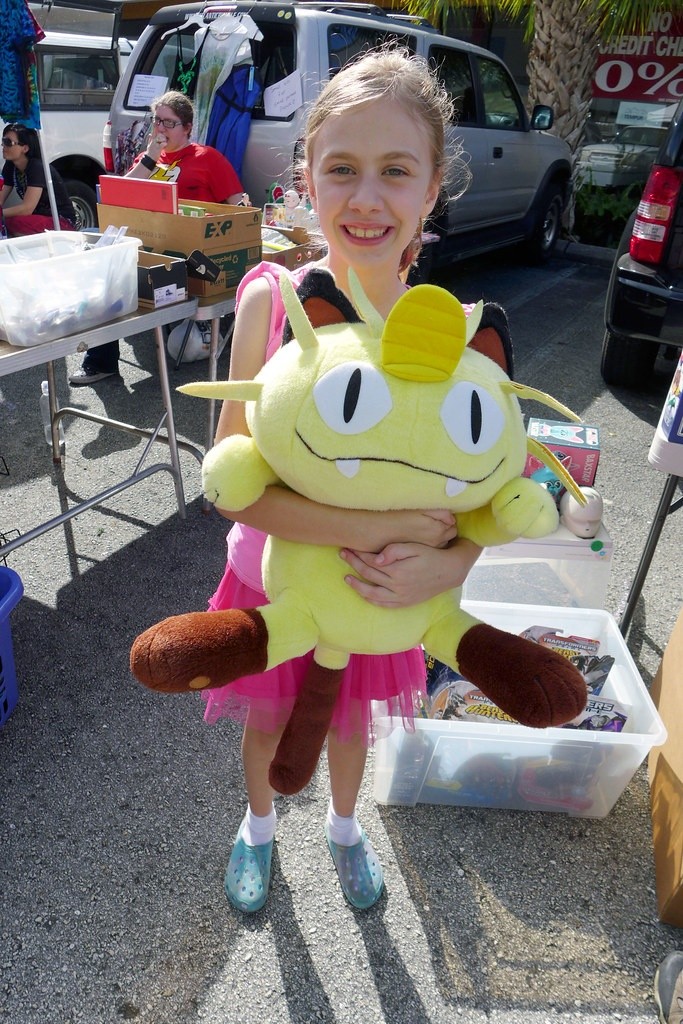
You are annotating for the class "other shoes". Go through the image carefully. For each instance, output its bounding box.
[324,823,384,909]
[69,365,119,383]
[225,817,274,912]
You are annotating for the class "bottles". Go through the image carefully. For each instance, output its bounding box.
[40,381,65,445]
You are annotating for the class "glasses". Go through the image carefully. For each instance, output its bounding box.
[2,137,20,147]
[150,115,183,128]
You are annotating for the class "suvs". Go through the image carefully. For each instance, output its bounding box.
[102,0,574,306]
[580,124,668,189]
[601,98,683,431]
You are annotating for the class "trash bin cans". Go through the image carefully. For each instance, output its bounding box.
[0,565,25,729]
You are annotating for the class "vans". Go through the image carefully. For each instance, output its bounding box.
[0,31,196,231]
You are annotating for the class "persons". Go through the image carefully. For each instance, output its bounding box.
[68,91,243,383]
[0,123,76,237]
[202,51,484,913]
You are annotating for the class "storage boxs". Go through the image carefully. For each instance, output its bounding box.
[0,566,24,726]
[365,417,683,926]
[1,198,320,348]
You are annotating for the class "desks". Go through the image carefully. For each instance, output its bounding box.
[0,293,198,562]
[137,231,440,515]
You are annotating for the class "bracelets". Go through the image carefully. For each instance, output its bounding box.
[139,153,157,171]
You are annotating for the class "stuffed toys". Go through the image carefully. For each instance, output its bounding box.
[131,268,587,795]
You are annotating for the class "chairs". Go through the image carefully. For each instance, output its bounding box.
[161,0,257,40]
